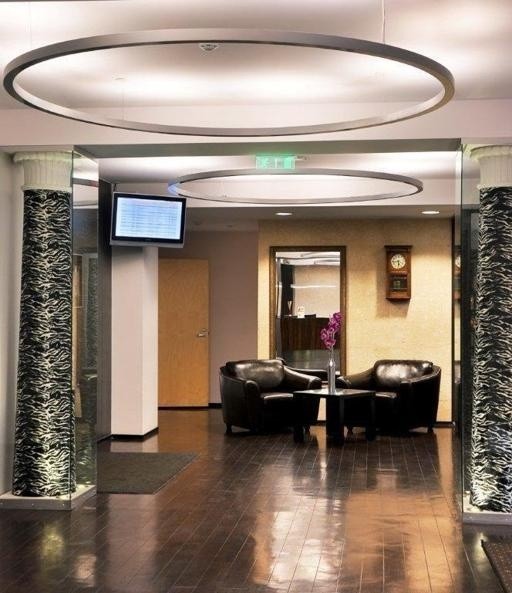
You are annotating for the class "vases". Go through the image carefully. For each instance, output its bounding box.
[326,352,335,392]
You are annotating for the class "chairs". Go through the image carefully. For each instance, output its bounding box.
[336,359,441,436]
[219,360,321,434]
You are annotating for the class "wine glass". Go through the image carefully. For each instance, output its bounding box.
[287,301,292,316]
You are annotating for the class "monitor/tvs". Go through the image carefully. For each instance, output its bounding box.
[109,192,186,248]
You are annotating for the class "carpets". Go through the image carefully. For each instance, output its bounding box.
[96,451,197,495]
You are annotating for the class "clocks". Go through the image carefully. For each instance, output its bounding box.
[384,244,413,300]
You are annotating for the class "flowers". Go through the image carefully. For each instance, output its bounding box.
[320,313,342,354]
